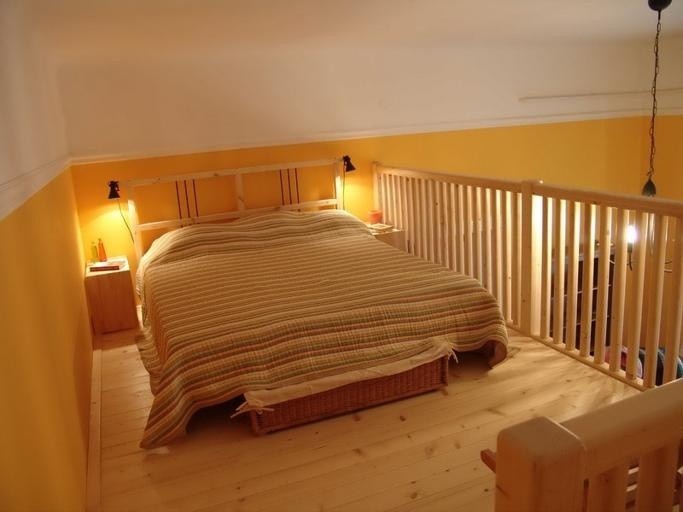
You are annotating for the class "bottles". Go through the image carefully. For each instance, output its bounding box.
[96,238,107,261]
[90,241,98,264]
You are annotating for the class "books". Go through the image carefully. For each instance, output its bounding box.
[368,223,393,233]
[90,261,125,272]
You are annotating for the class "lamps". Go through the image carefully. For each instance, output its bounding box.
[104,177,133,243]
[633,0,672,258]
[340,154,355,209]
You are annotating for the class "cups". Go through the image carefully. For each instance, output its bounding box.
[368,209,383,225]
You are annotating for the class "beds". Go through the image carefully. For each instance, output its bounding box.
[125,156,512,451]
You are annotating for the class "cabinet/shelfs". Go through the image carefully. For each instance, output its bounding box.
[550,240,616,354]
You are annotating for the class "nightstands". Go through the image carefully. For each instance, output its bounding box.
[83,254,140,336]
[367,221,407,251]
